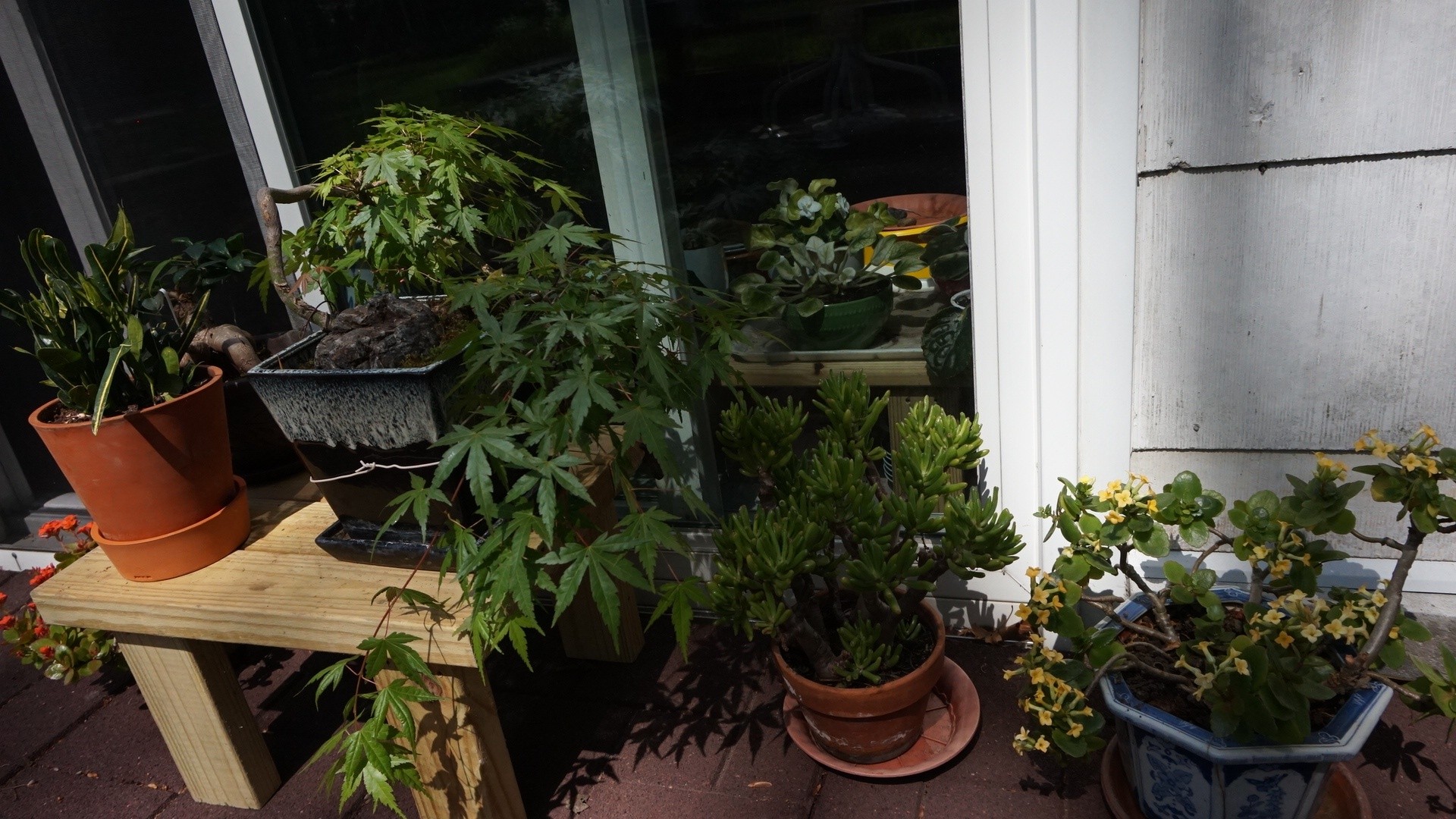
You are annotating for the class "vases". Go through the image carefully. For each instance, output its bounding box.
[1082,586,1394,819]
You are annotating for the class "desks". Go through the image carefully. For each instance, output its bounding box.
[28,424,647,819]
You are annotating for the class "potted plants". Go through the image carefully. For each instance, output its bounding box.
[0,99,1029,819]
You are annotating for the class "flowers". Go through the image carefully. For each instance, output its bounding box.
[0,514,131,685]
[1000,424,1456,755]
